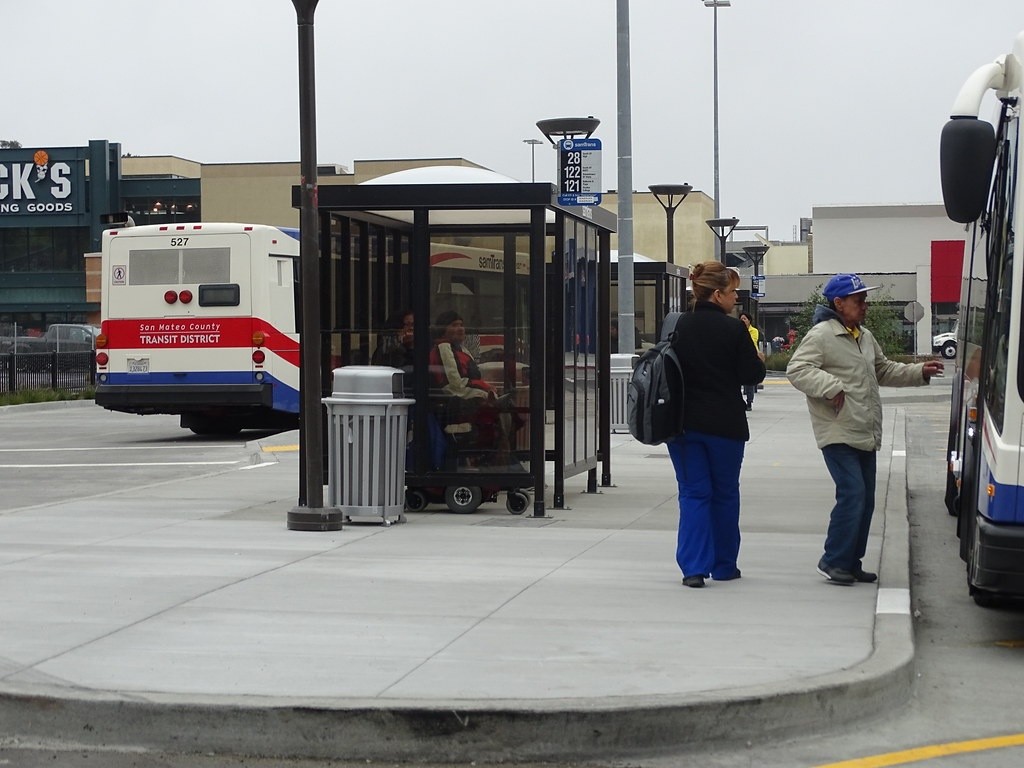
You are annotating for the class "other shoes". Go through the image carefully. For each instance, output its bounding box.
[746,406,752,411]
[736,568,741,577]
[683,575,705,586]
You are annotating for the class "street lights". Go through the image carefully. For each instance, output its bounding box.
[704,1,734,259]
[704,217,740,269]
[648,183,694,265]
[523,138,544,183]
[742,245,772,335]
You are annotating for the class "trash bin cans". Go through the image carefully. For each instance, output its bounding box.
[610,352,641,434]
[320,364,416,528]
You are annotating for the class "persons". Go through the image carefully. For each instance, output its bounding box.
[785,273,944,584]
[373,308,517,466]
[610,319,619,354]
[658,262,766,589]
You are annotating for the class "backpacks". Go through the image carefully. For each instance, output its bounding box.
[628,311,688,446]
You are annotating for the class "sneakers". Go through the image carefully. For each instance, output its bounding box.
[849,561,877,582]
[816,558,854,585]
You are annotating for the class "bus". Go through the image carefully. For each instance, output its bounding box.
[938,31,1024,610]
[91,221,529,437]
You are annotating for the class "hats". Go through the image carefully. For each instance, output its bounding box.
[822,274,880,302]
[436,311,463,337]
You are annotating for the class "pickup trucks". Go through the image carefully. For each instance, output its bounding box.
[0,324,102,375]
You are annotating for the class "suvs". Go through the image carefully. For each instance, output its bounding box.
[932,319,960,361]
